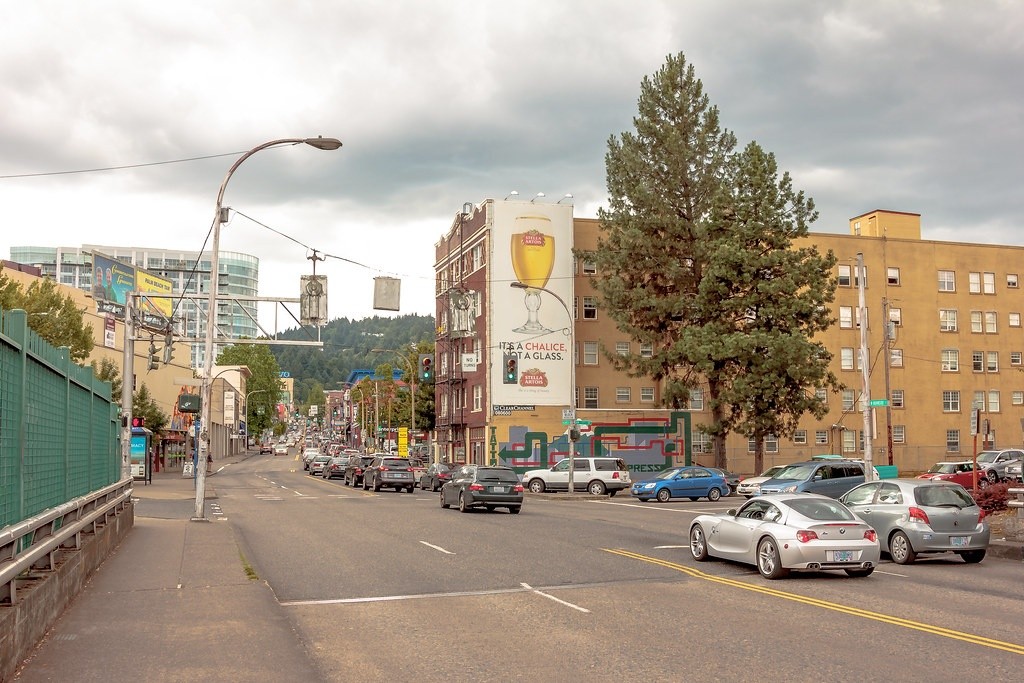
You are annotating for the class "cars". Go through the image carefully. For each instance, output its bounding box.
[420,464,464,492]
[1005,456,1022,479]
[735,465,789,498]
[386,446,430,463]
[279,419,373,487]
[916,461,988,490]
[837,478,989,564]
[687,469,744,497]
[630,466,728,501]
[410,459,430,485]
[273,444,288,455]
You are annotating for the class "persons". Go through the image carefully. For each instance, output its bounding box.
[94,267,117,301]
[206,452,213,472]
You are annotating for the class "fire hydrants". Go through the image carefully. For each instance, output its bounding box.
[206,455,214,472]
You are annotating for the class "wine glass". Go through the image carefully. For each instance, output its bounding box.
[508,216,557,335]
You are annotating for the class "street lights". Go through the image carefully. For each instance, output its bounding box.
[245,390,267,457]
[209,368,246,456]
[510,282,574,493]
[328,349,416,458]
[193,137,345,517]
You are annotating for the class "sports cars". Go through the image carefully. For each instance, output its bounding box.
[688,491,881,580]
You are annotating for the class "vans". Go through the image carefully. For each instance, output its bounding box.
[754,460,866,500]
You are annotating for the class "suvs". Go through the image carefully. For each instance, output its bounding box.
[965,449,1024,484]
[260,442,273,454]
[521,457,631,497]
[440,463,524,514]
[362,457,415,494]
[851,459,879,481]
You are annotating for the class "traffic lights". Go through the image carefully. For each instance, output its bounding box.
[571,430,580,438]
[148,344,161,371]
[419,354,435,381]
[164,328,175,365]
[503,355,518,384]
[133,417,144,427]
